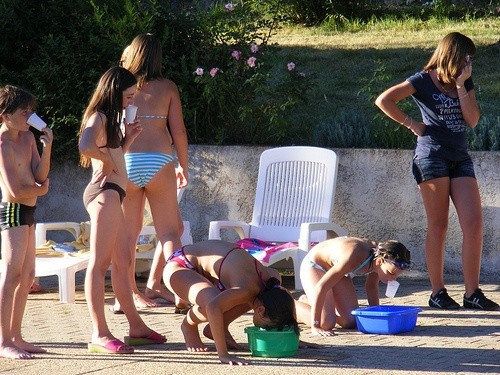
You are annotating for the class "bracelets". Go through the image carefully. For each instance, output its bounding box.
[457,84,468,99]
[408,118,413,129]
[400,116,408,125]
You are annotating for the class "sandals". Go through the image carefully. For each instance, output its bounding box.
[28,280,41,293]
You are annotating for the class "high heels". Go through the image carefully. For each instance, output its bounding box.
[124,330,168,344]
[87,336,134,355]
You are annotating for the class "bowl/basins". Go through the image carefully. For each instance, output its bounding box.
[244,324,301,357]
[351,302,422,334]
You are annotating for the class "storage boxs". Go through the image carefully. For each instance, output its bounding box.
[355,304,422,333]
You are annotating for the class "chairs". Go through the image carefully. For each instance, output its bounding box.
[209,145,347,294]
[33,165,189,305]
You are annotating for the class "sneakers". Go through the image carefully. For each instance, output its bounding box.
[463,288,500,310]
[428,289,460,310]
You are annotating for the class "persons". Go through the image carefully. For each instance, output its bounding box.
[375,33,499,311]
[78,66,168,354]
[0,84,53,358]
[294,236,411,336]
[119,45,175,306]
[163,240,323,365]
[114,32,189,311]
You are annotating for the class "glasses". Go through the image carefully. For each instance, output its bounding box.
[119,60,125,67]
[383,258,410,270]
[464,54,472,64]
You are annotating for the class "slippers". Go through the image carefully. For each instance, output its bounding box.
[175,303,193,314]
[112,304,125,313]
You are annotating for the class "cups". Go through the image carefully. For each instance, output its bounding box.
[125,104,138,125]
[26,112,47,132]
[385,280,400,298]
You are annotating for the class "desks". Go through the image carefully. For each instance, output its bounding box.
[135,219,195,260]
